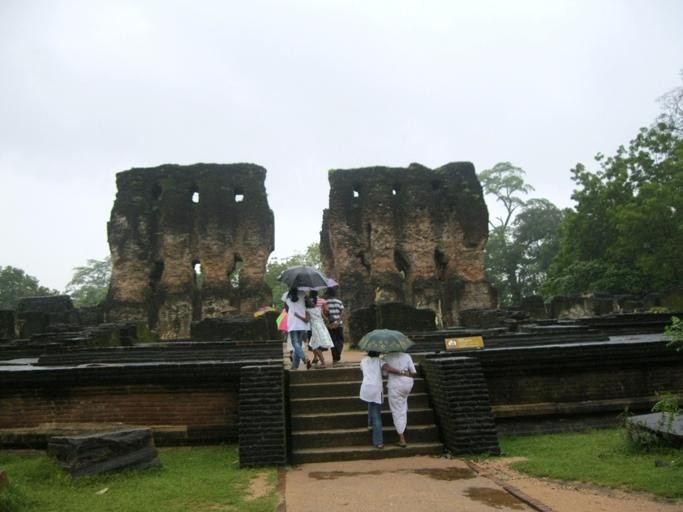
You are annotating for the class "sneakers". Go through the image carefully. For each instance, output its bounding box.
[312,360,326,369]
[332,361,343,367]
[367,424,406,448]
[307,361,311,369]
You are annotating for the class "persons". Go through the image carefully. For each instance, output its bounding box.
[280,286,313,370]
[320,287,344,368]
[379,351,417,448]
[307,289,327,365]
[357,351,411,450]
[285,330,305,362]
[294,296,334,370]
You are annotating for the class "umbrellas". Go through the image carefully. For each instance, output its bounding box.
[276,263,338,292]
[357,328,415,355]
[275,306,288,332]
